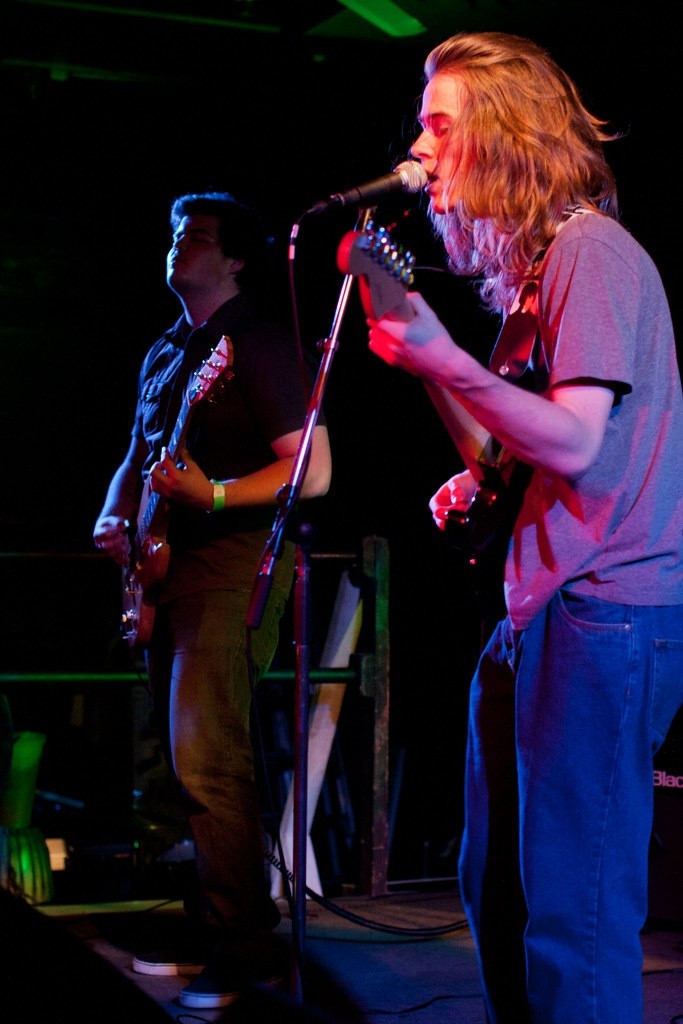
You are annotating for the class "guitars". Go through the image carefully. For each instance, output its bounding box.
[117,335,241,649]
[335,214,544,580]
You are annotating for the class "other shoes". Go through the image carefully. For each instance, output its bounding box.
[131,920,249,976]
[179,930,308,1010]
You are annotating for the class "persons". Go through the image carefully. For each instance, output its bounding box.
[87,185,339,1011]
[362,27,682,1022]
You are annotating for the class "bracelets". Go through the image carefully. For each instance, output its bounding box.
[210,478,226,515]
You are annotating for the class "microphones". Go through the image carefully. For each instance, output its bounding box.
[306,161,429,214]
